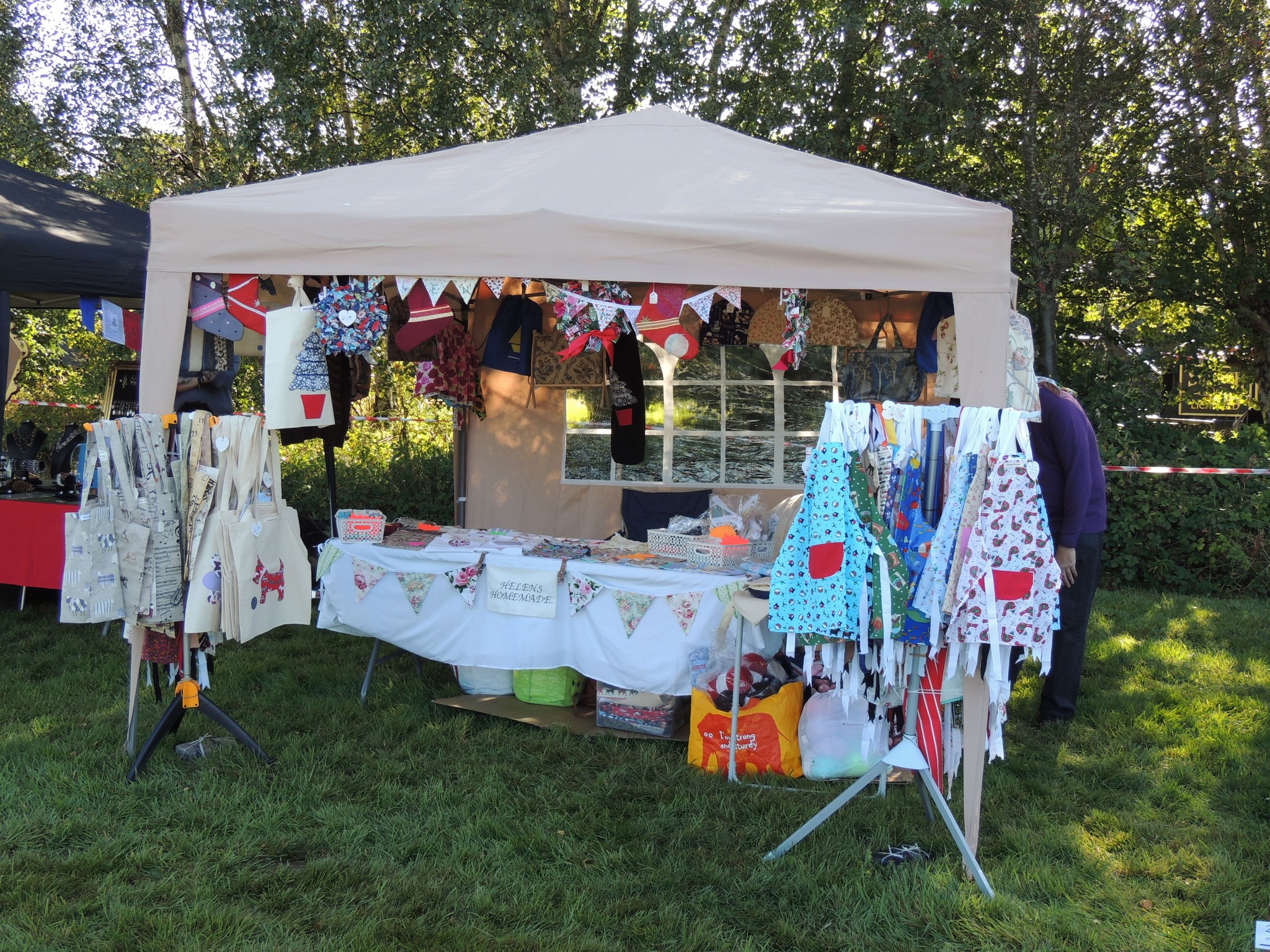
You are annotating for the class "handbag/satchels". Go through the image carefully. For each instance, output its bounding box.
[839,313,924,403]
[265,276,337,429]
[687,682,805,778]
[60,409,312,644]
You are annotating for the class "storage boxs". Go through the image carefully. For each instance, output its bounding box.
[686,538,753,570]
[646,527,707,560]
[76,444,98,489]
[596,681,687,738]
[751,540,775,559]
[335,508,389,540]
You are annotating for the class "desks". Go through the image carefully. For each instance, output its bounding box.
[0,493,87,610]
[317,519,762,698]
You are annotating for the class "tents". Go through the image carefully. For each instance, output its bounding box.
[0,155,337,541]
[124,105,1013,878]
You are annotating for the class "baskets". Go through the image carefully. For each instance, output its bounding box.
[337,510,385,542]
[647,523,776,566]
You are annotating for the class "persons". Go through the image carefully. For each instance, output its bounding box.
[6,419,48,461]
[981,377,1107,725]
[174,355,241,416]
[51,422,84,480]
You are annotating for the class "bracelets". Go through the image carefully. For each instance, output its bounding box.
[197,375,208,390]
[10,458,47,474]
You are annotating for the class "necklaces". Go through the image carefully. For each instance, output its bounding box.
[55,428,82,452]
[13,427,41,453]
